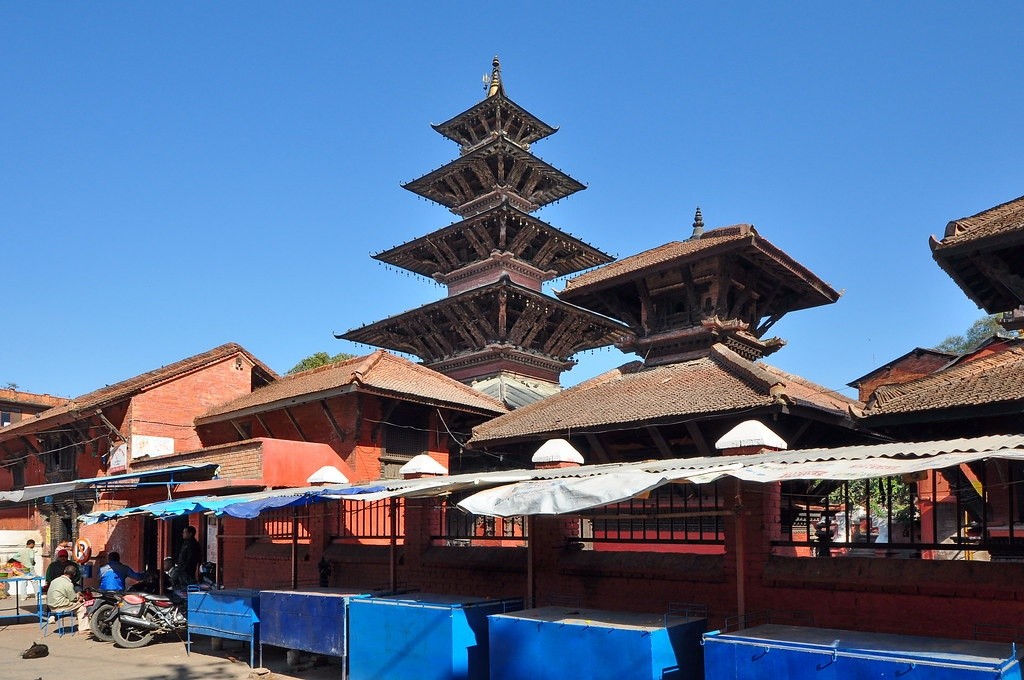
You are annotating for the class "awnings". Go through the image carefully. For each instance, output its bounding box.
[0,463,219,503]
[76,437,1024,518]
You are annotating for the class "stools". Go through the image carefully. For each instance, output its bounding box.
[45,612,75,637]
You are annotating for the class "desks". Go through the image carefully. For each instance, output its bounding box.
[186,585,1024,680]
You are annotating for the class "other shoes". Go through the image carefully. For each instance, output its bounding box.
[79,629,93,634]
[49,615,56,624]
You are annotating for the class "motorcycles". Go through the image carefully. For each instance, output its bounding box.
[102,561,225,649]
[82,557,180,641]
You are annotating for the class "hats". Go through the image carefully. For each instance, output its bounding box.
[58,549,68,557]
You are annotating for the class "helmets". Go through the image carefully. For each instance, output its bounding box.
[199,562,216,582]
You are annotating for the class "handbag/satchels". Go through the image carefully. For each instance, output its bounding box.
[26,580,35,594]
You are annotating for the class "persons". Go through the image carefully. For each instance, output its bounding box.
[163,556,191,596]
[47,565,92,635]
[178,526,202,585]
[54,537,74,560]
[97,552,147,591]
[13,539,42,600]
[46,549,80,623]
